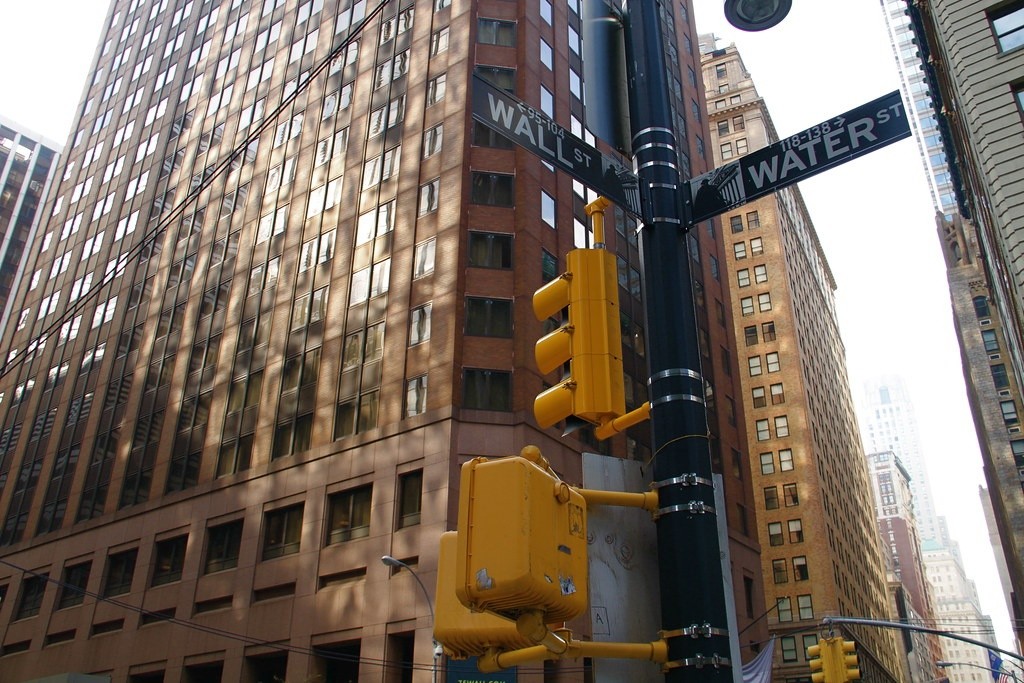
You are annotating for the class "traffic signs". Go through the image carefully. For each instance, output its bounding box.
[689,87,915,227]
[465,68,640,225]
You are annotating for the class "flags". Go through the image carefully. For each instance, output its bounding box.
[988,650,1008,683]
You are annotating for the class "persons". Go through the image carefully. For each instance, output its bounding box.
[693,179,727,216]
[604,164,627,205]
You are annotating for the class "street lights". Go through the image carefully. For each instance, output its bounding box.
[378,556,439,683]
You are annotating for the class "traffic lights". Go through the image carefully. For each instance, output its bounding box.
[833,637,863,683]
[533,247,627,433]
[807,638,836,683]
